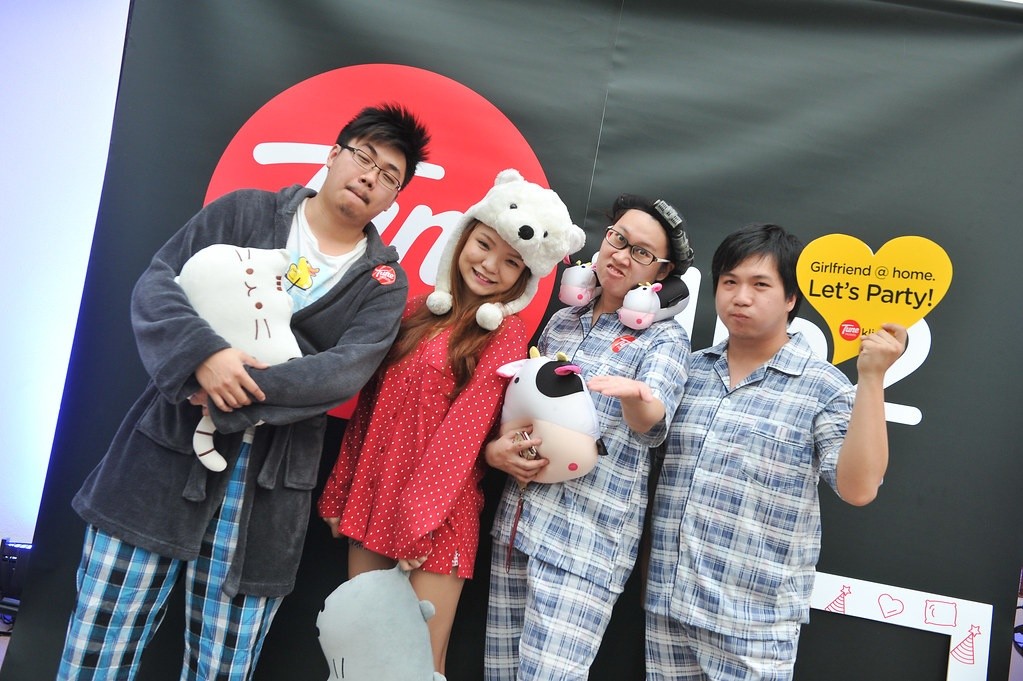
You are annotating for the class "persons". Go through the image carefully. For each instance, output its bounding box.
[57,101,909,681]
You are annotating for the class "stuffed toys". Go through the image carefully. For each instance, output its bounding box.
[495,346,609,484]
[172,244,305,473]
[315,562,447,681]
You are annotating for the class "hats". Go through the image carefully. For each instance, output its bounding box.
[427,168,588,328]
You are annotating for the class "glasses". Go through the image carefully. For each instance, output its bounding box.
[606,225,670,266]
[341,144,402,191]
